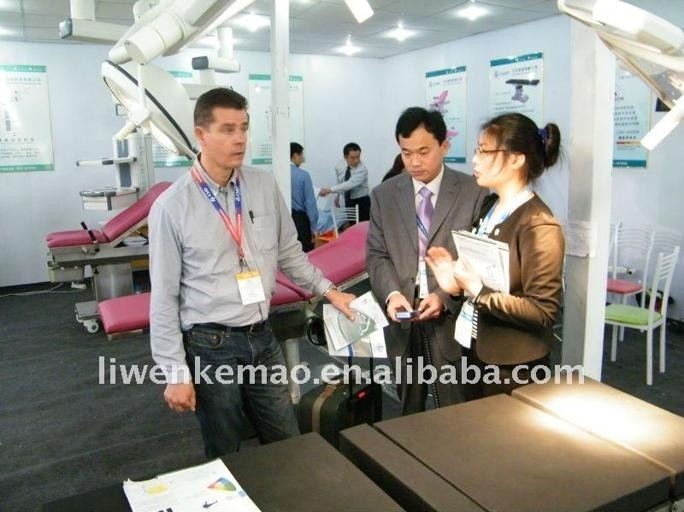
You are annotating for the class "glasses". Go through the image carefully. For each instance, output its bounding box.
[472,145,513,156]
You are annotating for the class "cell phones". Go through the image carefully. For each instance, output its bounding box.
[396,311,417,319]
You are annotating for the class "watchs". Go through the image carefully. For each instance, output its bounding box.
[323,283,338,300]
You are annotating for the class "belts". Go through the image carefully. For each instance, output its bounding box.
[195,310,279,334]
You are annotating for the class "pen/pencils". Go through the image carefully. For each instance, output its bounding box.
[249,210,255,224]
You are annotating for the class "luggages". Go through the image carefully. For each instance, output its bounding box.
[297,359,381,450]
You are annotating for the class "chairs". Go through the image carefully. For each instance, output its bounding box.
[604,217,682,387]
[328,204,360,240]
[43,177,173,248]
[94,218,373,342]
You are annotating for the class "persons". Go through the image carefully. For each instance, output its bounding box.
[424,112,566,399]
[380,153,404,182]
[291,142,319,254]
[319,142,371,226]
[149,87,357,462]
[365,107,490,417]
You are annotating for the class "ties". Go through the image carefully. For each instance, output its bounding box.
[344,167,350,201]
[416,187,433,263]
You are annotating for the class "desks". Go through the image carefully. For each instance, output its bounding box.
[39,367,682,512]
